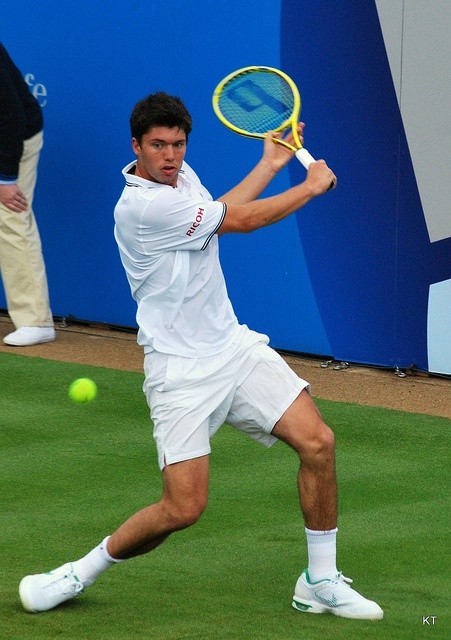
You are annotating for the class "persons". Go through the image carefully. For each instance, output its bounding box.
[18,91,384,621]
[0,42,56,346]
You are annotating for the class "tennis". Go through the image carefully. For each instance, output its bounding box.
[69,377,98,404]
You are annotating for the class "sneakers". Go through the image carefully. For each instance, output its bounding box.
[3,327,56,346]
[18,561,85,613]
[292,567,384,621]
